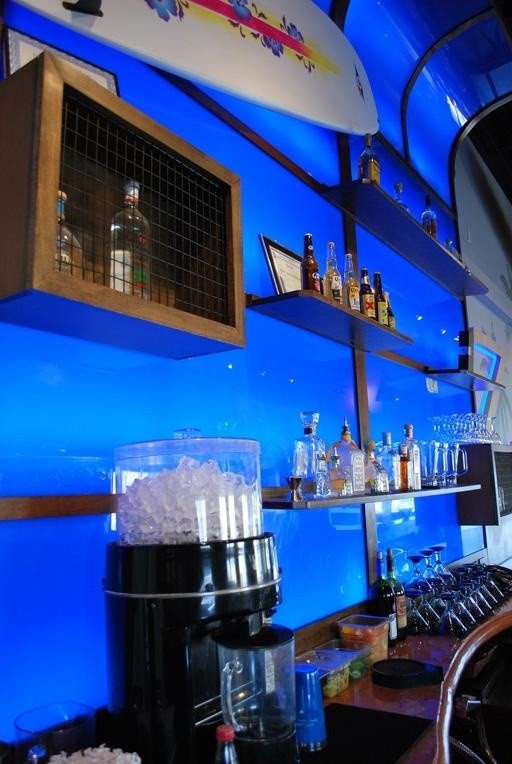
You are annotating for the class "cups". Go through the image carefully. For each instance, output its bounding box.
[14,700,96,764]
[286,477,303,501]
[422,441,468,487]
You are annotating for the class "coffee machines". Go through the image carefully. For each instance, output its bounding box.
[98,425,299,764]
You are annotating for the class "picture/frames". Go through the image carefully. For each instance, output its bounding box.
[469,327,507,426]
[1,21,121,100]
[257,233,305,295]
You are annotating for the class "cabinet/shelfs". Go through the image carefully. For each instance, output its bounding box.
[455,441,511,525]
[246,164,507,510]
[1,50,248,361]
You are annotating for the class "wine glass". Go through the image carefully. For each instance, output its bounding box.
[407,542,506,635]
[428,411,505,444]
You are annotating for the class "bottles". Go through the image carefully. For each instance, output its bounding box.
[363,439,389,493]
[375,432,398,491]
[358,133,381,185]
[392,181,411,215]
[369,550,399,645]
[384,547,408,640]
[398,424,422,490]
[328,446,354,497]
[57,190,84,280]
[421,194,438,239]
[328,416,363,497]
[109,180,152,301]
[323,241,343,306]
[384,291,395,329]
[444,238,462,260]
[344,252,362,312]
[374,272,389,325]
[288,411,328,499]
[361,267,375,319]
[299,233,321,295]
[214,724,240,763]
